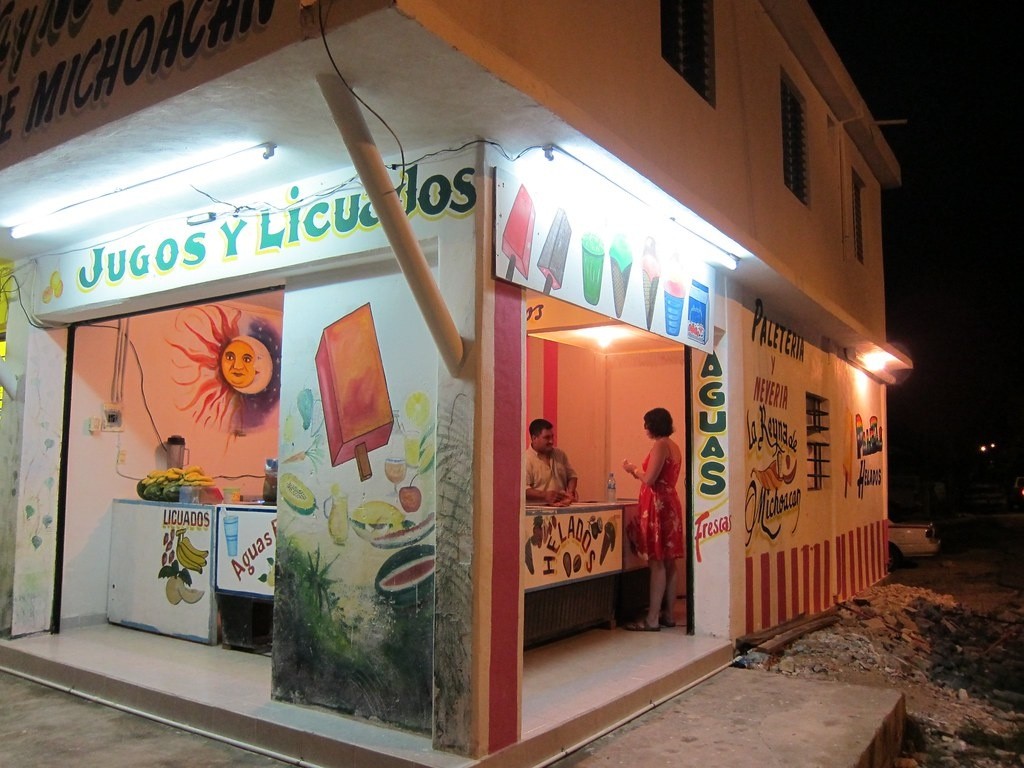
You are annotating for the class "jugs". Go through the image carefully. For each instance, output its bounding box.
[263,459,278,502]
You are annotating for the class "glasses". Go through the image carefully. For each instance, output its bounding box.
[644,423,650,429]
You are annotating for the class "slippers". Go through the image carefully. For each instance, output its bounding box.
[659,613,676,627]
[622,616,661,631]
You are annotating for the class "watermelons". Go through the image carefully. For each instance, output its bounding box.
[162,482,181,501]
[137,478,145,500]
[144,485,162,500]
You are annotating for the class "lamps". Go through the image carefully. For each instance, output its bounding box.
[847,347,897,384]
[543,144,744,272]
[8,141,278,240]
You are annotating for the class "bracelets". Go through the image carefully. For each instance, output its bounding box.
[634,468,640,476]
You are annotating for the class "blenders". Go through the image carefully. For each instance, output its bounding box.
[166,435,190,470]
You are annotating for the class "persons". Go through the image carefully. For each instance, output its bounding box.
[622,407,684,632]
[526,419,577,504]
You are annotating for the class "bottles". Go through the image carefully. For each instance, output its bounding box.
[179,483,193,503]
[606,472,617,504]
[198,481,223,504]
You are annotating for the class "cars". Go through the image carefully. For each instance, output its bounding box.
[1007,476,1024,509]
[886,517,942,573]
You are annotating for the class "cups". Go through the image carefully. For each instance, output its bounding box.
[223,486,240,504]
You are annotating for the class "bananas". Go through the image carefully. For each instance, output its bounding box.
[142,465,216,486]
